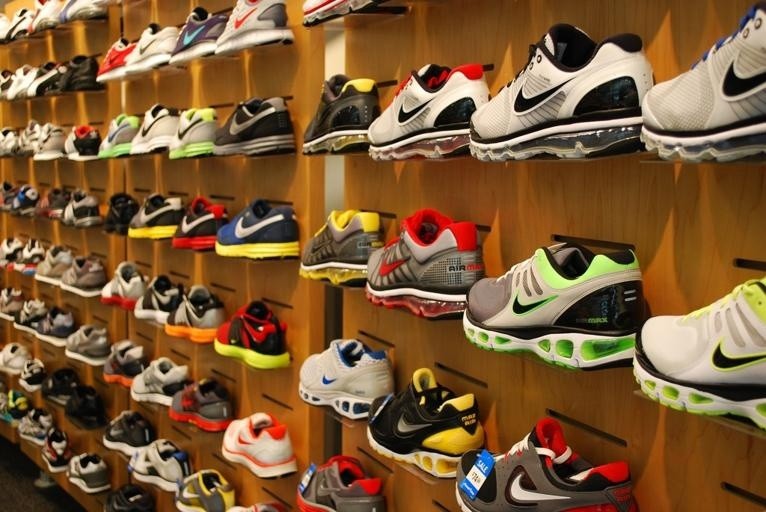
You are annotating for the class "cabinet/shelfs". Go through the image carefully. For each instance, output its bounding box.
[0,0,123,511]
[119,0,330,511]
[338,0,765,512]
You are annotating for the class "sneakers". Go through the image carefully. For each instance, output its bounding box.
[213,95,297,157]
[61,255,109,298]
[303,0,375,28]
[0,389,29,428]
[169,108,217,161]
[640,5,765,162]
[98,113,139,160]
[41,428,72,472]
[12,120,40,157]
[171,194,228,250]
[127,193,185,240]
[462,243,650,373]
[36,306,74,348]
[215,301,290,370]
[33,123,66,161]
[297,456,386,512]
[20,409,53,447]
[42,368,79,406]
[64,384,107,431]
[60,55,98,92]
[175,469,235,512]
[302,75,380,155]
[63,189,102,228]
[103,410,155,457]
[455,417,637,512]
[60,0,110,24]
[0,9,33,43]
[367,64,495,161]
[133,274,184,325]
[0,236,23,271]
[165,285,226,344]
[1,70,15,99]
[128,438,193,492]
[366,368,484,480]
[129,104,181,156]
[66,453,112,494]
[222,412,298,480]
[125,23,178,73]
[0,287,25,322]
[215,198,300,261]
[1,181,19,210]
[168,378,234,432]
[30,1,60,36]
[18,359,45,393]
[64,324,112,367]
[64,125,102,162]
[364,209,486,320]
[300,209,384,287]
[105,484,156,511]
[0,128,18,157]
[468,22,654,162]
[35,188,70,219]
[34,245,74,286]
[101,261,146,311]
[102,340,146,387]
[13,184,39,215]
[631,278,765,430]
[168,7,227,65]
[27,62,66,97]
[215,0,295,55]
[14,298,48,335]
[298,338,394,420]
[130,356,190,407]
[8,64,41,101]
[95,38,136,82]
[0,342,32,374]
[104,193,140,235]
[15,237,45,276]
